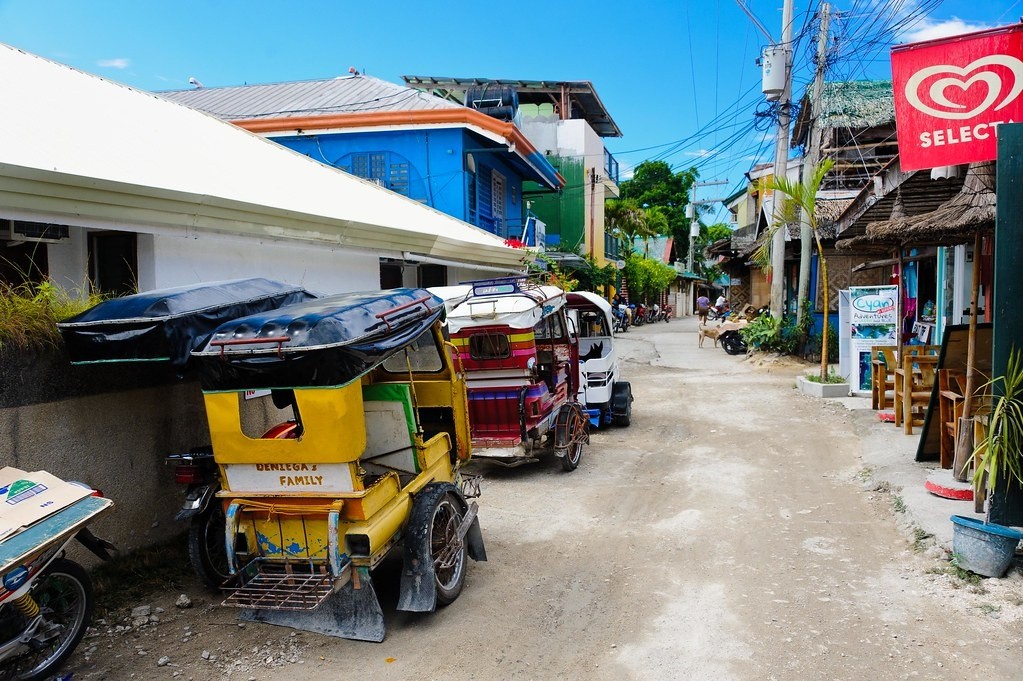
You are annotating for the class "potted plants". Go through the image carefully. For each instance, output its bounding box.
[951,344,1023,577]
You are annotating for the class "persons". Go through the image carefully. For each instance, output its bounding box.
[696,293,711,326]
[614,294,631,324]
[715,293,726,306]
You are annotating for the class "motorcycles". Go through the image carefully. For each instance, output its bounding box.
[717,300,788,355]
[706,302,740,321]
[612,301,635,333]
[635,303,672,326]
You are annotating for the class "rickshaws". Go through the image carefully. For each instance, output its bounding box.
[55,271,632,643]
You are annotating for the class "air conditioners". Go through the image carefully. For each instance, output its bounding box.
[0,219,71,245]
[384,258,421,267]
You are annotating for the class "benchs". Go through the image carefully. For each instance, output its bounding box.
[872,345,990,513]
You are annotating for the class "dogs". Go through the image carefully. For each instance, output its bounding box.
[698,322,720,348]
